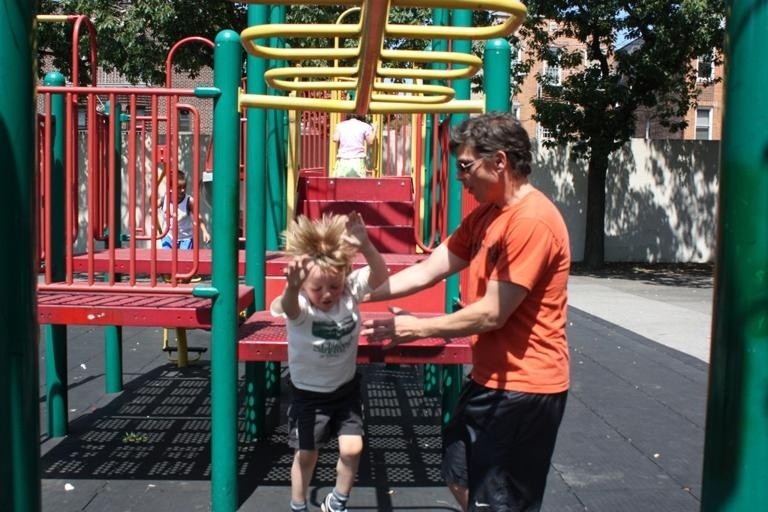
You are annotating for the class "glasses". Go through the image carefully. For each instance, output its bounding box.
[455,148,508,173]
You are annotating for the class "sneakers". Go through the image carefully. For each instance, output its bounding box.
[320,493,348,512]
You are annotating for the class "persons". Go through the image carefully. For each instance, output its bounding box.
[361,111,570,511]
[149,170,211,281]
[271,208,389,512]
[333,112,376,179]
